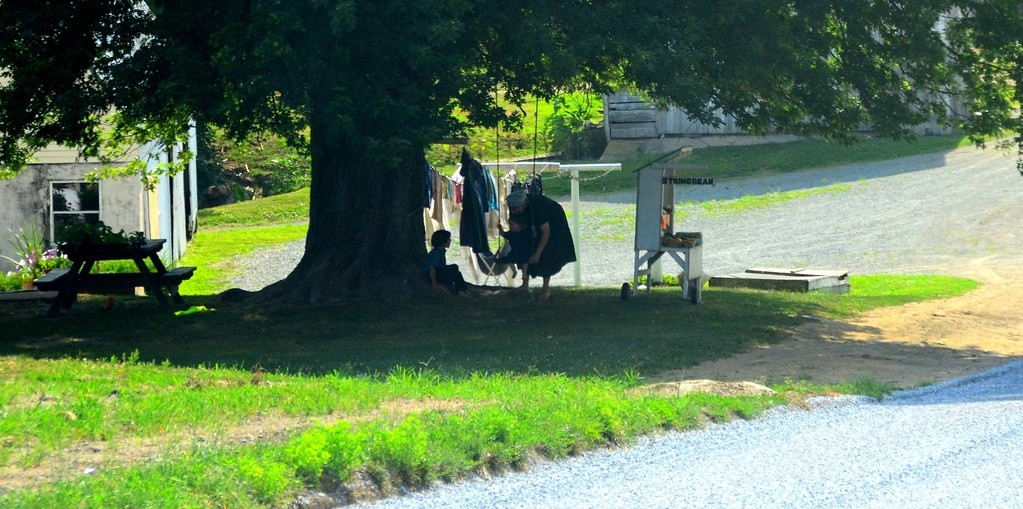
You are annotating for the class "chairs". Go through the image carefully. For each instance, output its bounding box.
[476,247,513,288]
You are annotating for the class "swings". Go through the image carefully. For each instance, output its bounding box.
[492,85,541,264]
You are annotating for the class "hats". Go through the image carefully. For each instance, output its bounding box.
[506,189,526,205]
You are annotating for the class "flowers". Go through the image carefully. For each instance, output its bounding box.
[22,274,33,282]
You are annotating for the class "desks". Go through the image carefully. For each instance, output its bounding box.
[47,239,182,317]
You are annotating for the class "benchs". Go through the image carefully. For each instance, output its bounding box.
[164,266,197,282]
[31,268,73,291]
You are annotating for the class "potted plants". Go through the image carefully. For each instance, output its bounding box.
[56,203,148,258]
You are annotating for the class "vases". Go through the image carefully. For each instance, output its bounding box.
[22,281,32,290]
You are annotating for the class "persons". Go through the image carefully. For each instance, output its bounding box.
[505,190,577,301]
[422,230,479,299]
[498,214,538,278]
